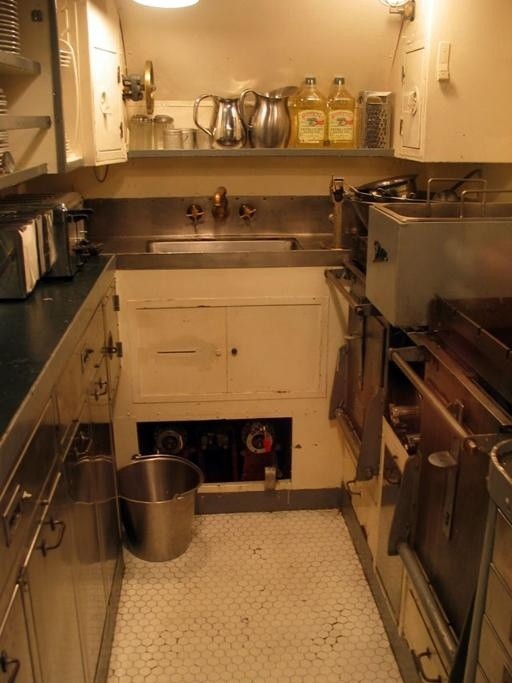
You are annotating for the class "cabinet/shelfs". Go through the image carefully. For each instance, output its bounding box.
[343,259,512,682]
[388,0,510,177]
[0,0,129,192]
[0,250,129,681]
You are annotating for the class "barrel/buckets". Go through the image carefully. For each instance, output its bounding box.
[117,452,205,563]
[71,452,118,564]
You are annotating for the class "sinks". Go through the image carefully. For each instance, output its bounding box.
[145,238,302,254]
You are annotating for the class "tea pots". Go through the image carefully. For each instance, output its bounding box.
[193,84,293,150]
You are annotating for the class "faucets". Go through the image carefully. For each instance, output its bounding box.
[213,186,228,219]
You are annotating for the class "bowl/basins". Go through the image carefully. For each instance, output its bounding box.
[355,174,419,199]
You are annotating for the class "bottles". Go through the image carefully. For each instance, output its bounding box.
[127,112,212,150]
[289,72,358,150]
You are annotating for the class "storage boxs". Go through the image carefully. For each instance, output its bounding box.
[361,177,512,330]
